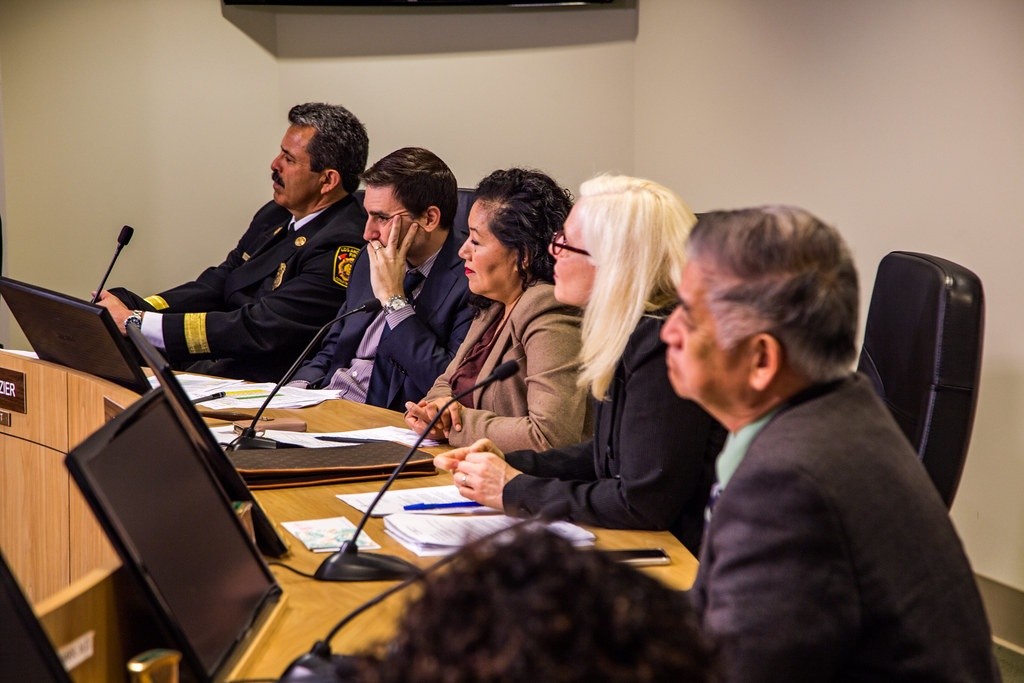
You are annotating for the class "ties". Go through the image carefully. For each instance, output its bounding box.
[287,222,295,238]
[365,271,426,409]
[705,481,724,527]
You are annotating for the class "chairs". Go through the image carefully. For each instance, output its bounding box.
[857,249,986,516]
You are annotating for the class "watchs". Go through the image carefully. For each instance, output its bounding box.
[383,294,408,315]
[124,310,143,333]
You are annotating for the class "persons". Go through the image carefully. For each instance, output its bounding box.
[91,102,369,384]
[405,168,599,450]
[659,204,1002,683]
[433,173,729,557]
[285,146,477,413]
[351,524,718,683]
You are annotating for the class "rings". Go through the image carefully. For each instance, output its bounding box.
[375,246,382,253]
[462,475,466,485]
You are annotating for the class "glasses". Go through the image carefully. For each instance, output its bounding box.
[551,230,592,257]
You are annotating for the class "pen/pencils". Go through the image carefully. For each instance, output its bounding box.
[403,501,485,510]
[191,391,226,405]
[315,436,389,443]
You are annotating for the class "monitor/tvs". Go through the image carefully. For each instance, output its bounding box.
[0,275,288,683]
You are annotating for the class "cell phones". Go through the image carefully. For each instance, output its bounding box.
[602,547,672,566]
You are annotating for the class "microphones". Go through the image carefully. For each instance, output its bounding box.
[226,299,382,452]
[92,225,134,304]
[314,359,520,581]
[277,496,574,681]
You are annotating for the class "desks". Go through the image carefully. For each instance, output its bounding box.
[1,348,704,683]
